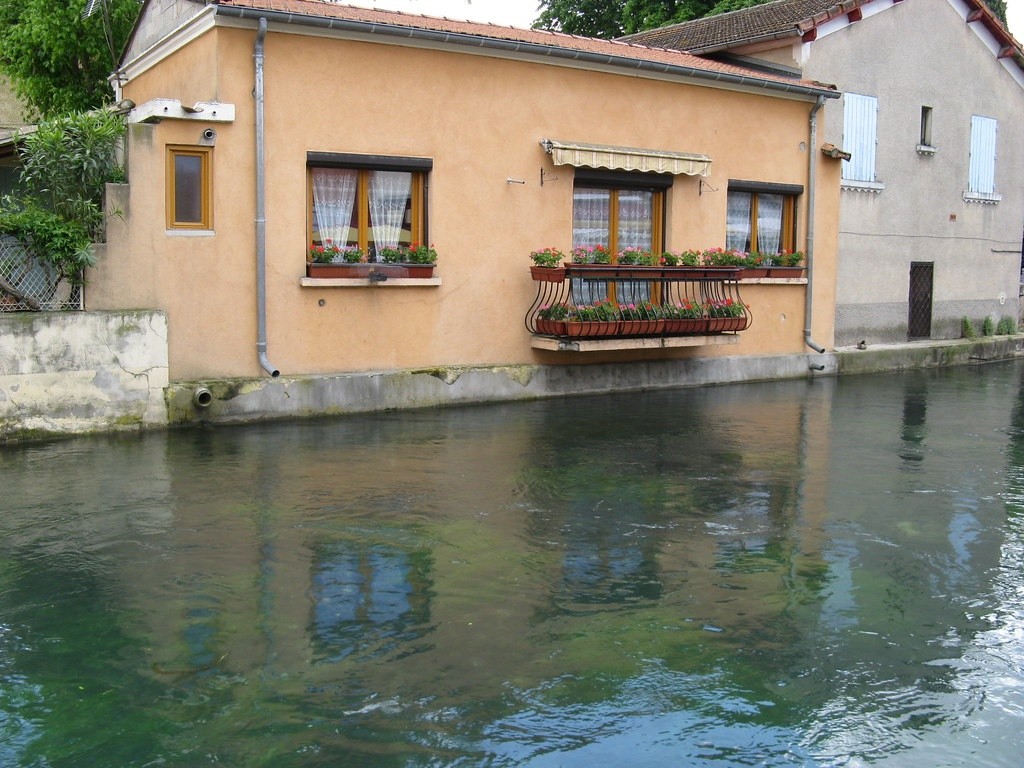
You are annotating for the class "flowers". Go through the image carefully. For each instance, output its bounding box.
[307,242,439,263]
[539,298,749,319]
[530,244,808,267]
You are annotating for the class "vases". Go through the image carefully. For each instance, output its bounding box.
[530,266,807,281]
[307,263,437,278]
[535,319,744,337]
